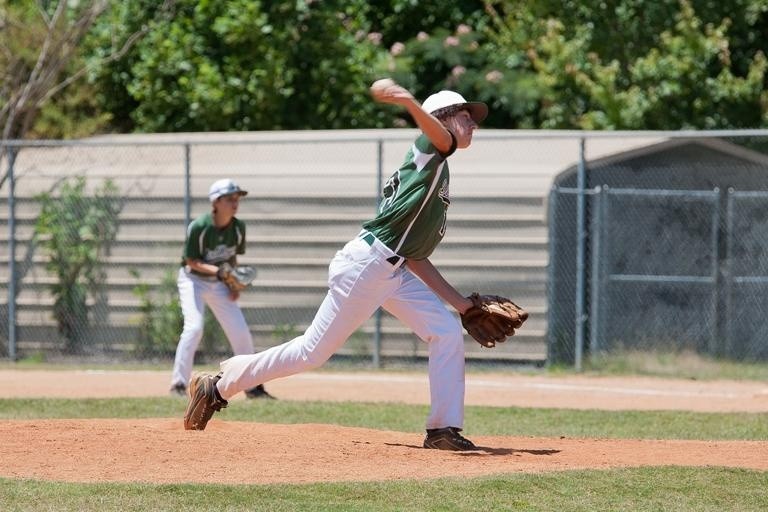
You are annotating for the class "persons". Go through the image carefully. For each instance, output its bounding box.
[183,86,528,451]
[167,180,278,401]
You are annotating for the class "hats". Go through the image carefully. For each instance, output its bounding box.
[208,177,249,204]
[421,90,489,124]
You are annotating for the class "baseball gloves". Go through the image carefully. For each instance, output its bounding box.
[460,291,528,348]
[216,263,256,299]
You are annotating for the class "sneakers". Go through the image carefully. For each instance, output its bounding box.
[184,372,228,431]
[245,383,278,400]
[424,427,476,447]
[170,382,189,397]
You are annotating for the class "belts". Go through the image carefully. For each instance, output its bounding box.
[360,230,408,269]
